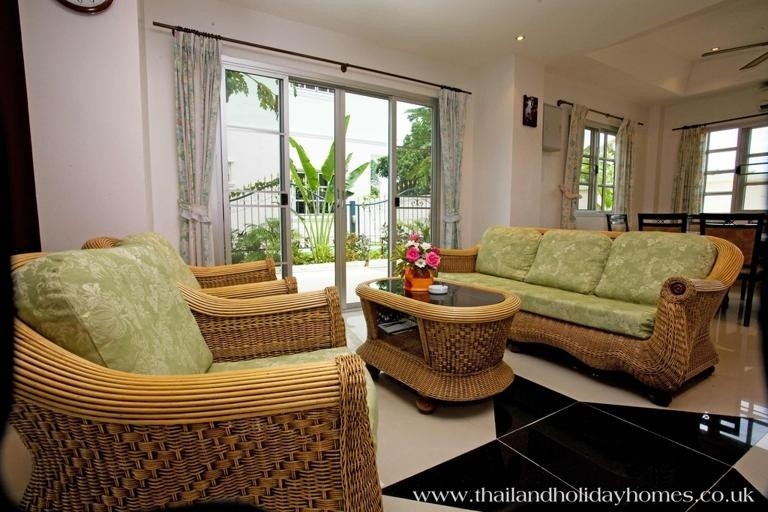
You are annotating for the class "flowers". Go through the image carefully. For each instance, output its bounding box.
[391,235,442,280]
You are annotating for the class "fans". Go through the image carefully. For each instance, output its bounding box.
[700,33,768,71]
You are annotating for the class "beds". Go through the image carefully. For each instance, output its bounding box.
[356,276,520,417]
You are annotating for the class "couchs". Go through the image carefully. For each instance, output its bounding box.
[429,224,745,406]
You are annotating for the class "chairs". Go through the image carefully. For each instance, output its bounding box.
[5,231,382,512]
[608,206,767,329]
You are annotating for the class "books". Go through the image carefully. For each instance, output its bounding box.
[378,318,418,335]
